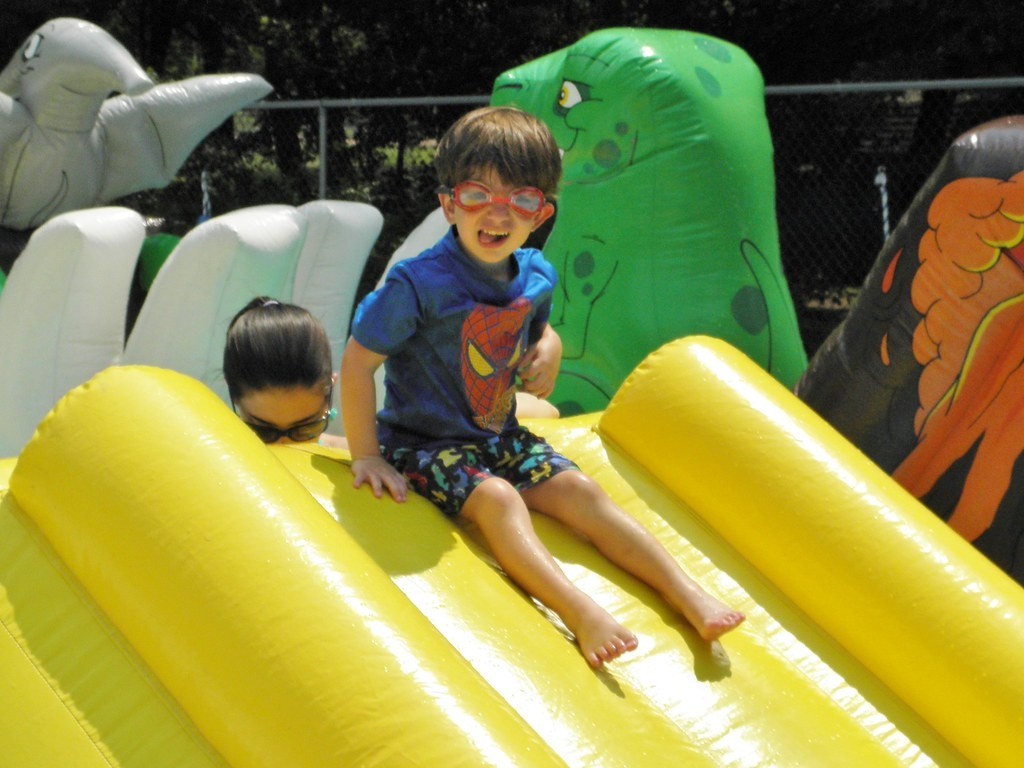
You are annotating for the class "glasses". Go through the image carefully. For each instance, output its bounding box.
[433,180,546,218]
[231,384,332,445]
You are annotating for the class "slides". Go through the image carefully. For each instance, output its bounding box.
[0,338,1023,767]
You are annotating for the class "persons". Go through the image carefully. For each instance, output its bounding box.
[224,295,337,445]
[339,104,746,665]
